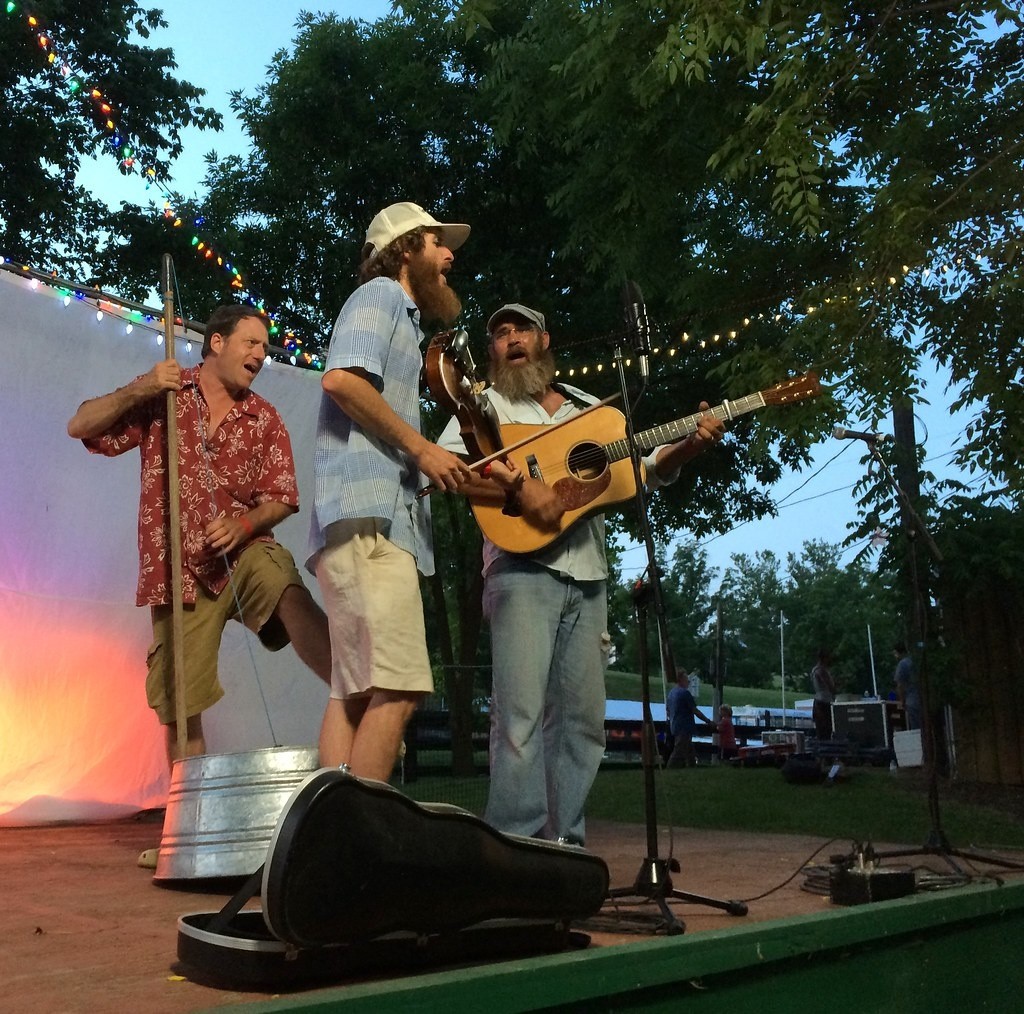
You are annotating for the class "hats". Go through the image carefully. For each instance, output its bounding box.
[365,201,471,260]
[486,303,545,333]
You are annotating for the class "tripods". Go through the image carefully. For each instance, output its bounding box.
[830,441,1024,872]
[601,341,749,936]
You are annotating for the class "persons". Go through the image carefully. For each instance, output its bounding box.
[435,303,726,846]
[811,650,838,741]
[68,304,331,866]
[304,202,526,783]
[714,704,735,760]
[894,642,922,730]
[665,668,715,769]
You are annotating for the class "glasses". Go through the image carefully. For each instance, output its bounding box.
[492,325,539,340]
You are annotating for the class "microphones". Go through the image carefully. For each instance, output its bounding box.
[623,280,652,385]
[833,427,893,443]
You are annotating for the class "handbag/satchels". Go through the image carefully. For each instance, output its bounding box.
[781,753,822,785]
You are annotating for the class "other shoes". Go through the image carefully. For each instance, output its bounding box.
[139,847,159,866]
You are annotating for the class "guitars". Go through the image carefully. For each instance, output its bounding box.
[469,371,822,555]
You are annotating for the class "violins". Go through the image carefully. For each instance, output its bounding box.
[424,323,521,516]
